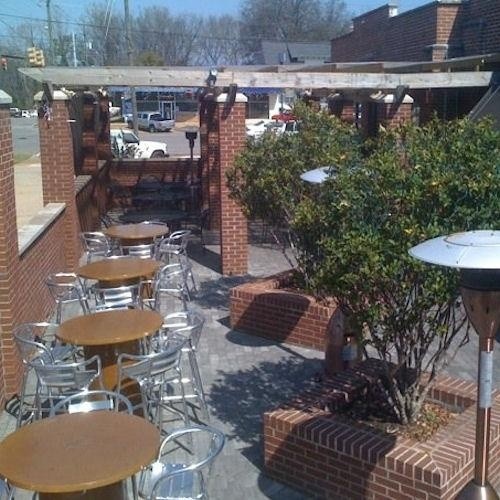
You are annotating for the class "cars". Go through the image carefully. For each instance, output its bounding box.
[245,118,305,140]
[10,107,38,118]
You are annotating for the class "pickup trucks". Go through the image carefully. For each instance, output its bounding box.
[128,111,175,133]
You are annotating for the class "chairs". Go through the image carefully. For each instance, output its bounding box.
[95,179,218,257]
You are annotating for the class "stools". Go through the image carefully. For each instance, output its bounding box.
[138,426,225,499]
[136,262,190,315]
[152,310,212,423]
[45,273,91,326]
[48,389,139,499]
[77,231,113,286]
[89,281,141,314]
[115,331,195,457]
[155,229,197,300]
[13,321,81,429]
[28,354,107,419]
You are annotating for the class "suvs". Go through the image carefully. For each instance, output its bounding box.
[110,129,169,159]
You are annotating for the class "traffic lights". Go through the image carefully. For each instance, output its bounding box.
[27,47,45,67]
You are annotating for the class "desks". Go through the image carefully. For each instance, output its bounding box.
[55,309,164,409]
[0,410,162,500]
[76,256,161,311]
